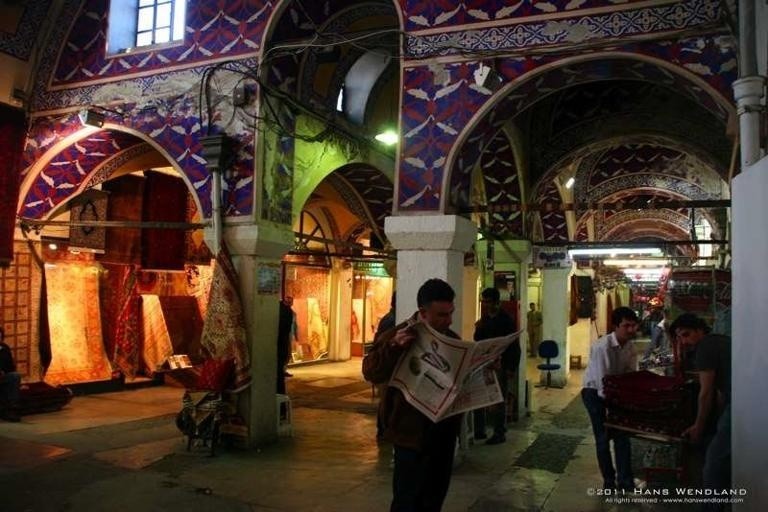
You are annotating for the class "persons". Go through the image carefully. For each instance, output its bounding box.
[527,302,543,358]
[643,315,668,367]
[667,312,732,510]
[283,295,299,378]
[507,282,515,301]
[580,306,645,498]
[0,326,21,423]
[372,291,395,443]
[361,277,503,511]
[419,339,450,374]
[474,286,522,446]
[276,298,293,421]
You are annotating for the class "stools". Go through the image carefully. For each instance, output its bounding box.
[276,393,293,441]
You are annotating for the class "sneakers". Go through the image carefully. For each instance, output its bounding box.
[483,427,509,444]
[603,481,639,494]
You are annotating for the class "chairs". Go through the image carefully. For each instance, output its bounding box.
[534,340,563,388]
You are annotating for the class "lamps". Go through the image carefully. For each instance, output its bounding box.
[558,175,575,189]
